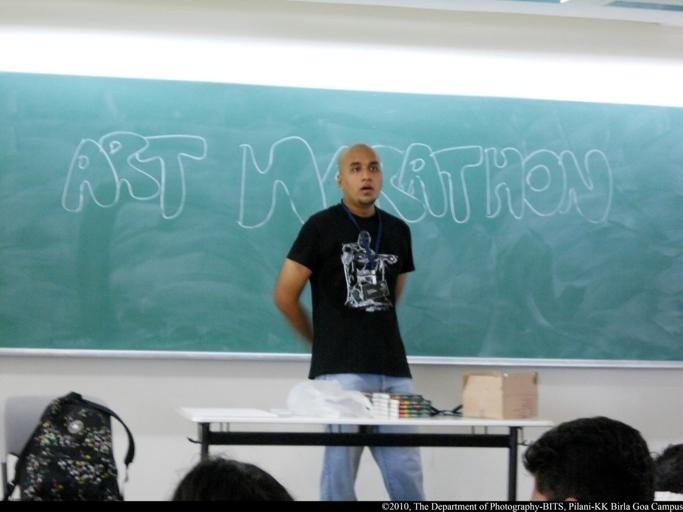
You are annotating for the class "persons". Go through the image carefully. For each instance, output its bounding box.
[271,142,427,501]
[170,457,296,502]
[521,416,655,508]
[653,440,683,506]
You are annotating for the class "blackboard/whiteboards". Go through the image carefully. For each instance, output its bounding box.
[0,71,683,369]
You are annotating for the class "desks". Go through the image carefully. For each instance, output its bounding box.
[178,406,555,506]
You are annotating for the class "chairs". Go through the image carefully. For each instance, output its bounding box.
[0,396,66,500]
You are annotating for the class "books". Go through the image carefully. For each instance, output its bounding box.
[367,391,431,419]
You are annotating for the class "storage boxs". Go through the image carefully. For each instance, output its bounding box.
[460,369,539,420]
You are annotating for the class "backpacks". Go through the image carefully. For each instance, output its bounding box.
[18,392,123,501]
